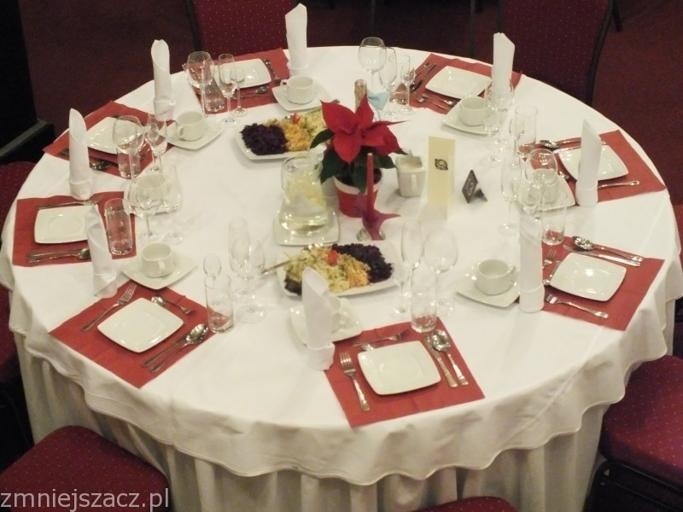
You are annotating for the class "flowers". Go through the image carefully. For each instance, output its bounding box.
[307,92,409,193]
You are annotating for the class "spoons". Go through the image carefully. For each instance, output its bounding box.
[431,327,471,389]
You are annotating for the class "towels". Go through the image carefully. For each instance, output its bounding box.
[517,207,546,313]
[149,37,173,125]
[83,199,116,300]
[490,31,516,113]
[298,263,333,375]
[575,116,601,210]
[63,104,91,203]
[282,4,308,81]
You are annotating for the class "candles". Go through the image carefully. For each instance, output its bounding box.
[367,153,374,211]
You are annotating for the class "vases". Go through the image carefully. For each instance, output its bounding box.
[332,168,382,218]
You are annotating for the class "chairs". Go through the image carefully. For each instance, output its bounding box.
[367,1,479,57]
[495,1,621,107]
[185,1,298,61]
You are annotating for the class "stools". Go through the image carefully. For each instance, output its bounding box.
[0,286,18,392]
[0,418,178,511]
[584,336,683,512]
[0,159,39,234]
[403,493,521,510]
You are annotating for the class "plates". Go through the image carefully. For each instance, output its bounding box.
[356,338,441,396]
[24,36,645,376]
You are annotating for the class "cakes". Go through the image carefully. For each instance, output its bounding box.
[285,240,395,298]
[239,109,334,156]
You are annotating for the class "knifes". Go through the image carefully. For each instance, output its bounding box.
[422,333,458,391]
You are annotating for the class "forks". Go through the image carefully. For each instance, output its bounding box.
[335,349,371,416]
